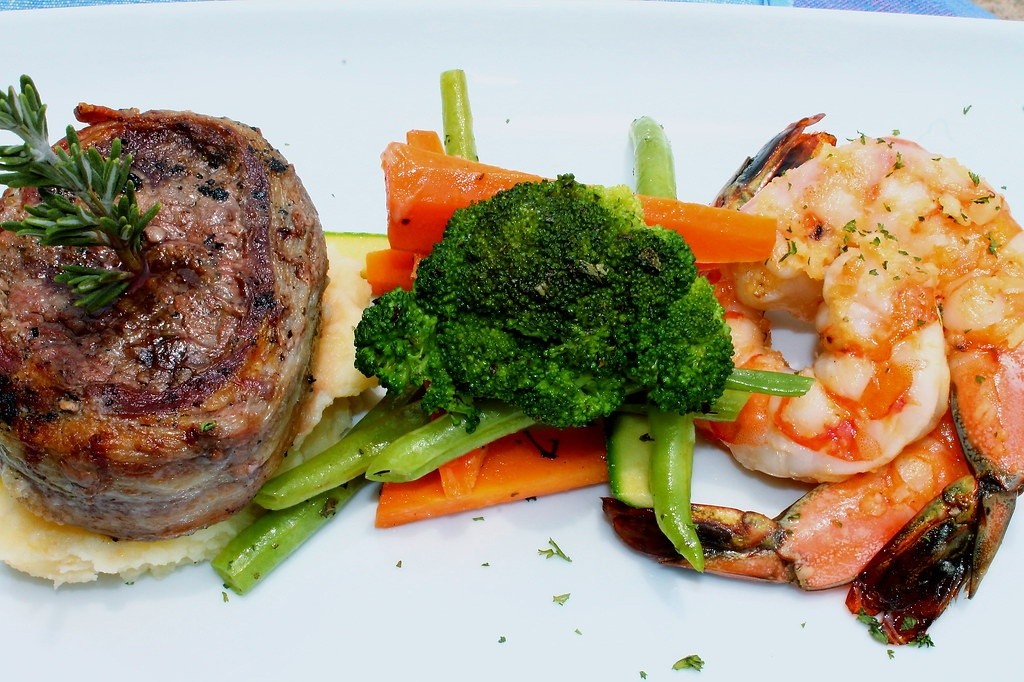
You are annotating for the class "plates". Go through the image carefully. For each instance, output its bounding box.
[2,0,1018,680]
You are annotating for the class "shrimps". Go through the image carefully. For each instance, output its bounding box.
[600,111,1023,641]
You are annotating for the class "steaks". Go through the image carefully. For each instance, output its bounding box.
[0,99,336,540]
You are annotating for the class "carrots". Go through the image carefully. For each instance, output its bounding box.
[362,128,787,299]
[371,418,611,537]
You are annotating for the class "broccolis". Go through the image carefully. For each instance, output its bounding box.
[351,172,732,431]
